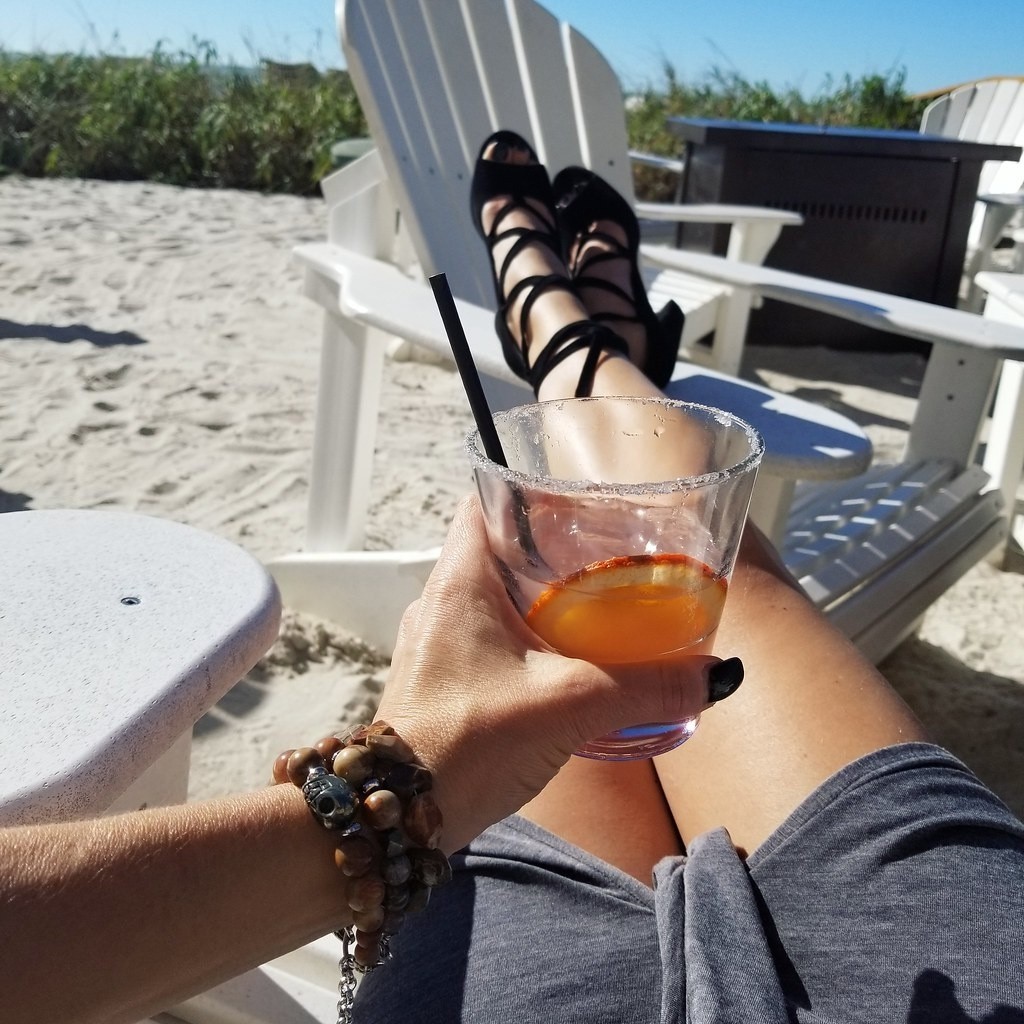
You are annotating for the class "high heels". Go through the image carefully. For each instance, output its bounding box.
[469,129,633,400]
[552,165,685,395]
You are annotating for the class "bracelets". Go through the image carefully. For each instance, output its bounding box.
[274,719,451,1024]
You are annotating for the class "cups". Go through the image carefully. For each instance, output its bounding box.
[467,398,764,764]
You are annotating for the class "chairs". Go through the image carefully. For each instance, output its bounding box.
[263,0,1024,671]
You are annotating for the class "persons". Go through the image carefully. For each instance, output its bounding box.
[0,130,1024,1024]
